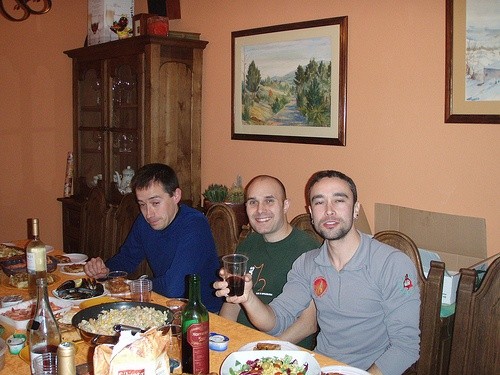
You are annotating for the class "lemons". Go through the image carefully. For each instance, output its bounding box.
[74,279,82,287]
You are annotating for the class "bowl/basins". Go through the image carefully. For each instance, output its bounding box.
[71,300,174,348]
[1,258,28,277]
[47,255,57,273]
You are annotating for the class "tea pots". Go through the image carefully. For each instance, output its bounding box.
[114,166,135,195]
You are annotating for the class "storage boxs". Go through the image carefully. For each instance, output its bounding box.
[87,0,134,46]
[356,202,488,318]
[129,0,181,37]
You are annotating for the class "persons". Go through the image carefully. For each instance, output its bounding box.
[220,175,321,351]
[84,164,226,314]
[214,170,421,375]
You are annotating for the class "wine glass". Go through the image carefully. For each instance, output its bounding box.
[92,78,136,107]
[96,132,134,152]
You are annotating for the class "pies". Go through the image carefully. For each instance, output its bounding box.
[54,255,72,263]
[9,273,55,288]
[105,277,130,293]
[253,343,281,350]
[0,244,25,257]
[64,264,85,272]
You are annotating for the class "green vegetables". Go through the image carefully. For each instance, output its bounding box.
[230,355,307,375]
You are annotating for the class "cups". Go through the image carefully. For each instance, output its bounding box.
[94,174,122,183]
[157,325,181,362]
[165,298,189,325]
[129,280,152,303]
[221,254,248,297]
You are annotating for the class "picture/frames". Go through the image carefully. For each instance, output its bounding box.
[443,0,500,125]
[230,16,348,145]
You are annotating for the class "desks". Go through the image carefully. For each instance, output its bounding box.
[0,241,370,375]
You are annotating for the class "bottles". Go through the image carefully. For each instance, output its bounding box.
[29,278,61,375]
[181,274,210,375]
[26,218,47,297]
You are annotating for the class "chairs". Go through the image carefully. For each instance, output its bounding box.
[447,257,500,375]
[205,205,324,265]
[371,230,450,375]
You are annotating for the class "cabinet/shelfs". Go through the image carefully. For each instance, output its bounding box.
[57,34,208,281]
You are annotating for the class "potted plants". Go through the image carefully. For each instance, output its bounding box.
[201,183,249,234]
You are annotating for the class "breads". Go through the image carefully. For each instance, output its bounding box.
[92,330,170,375]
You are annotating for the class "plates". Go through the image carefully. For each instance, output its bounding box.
[220,340,372,375]
[0,296,71,330]
[0,240,133,304]
[0,320,17,341]
[18,341,79,365]
